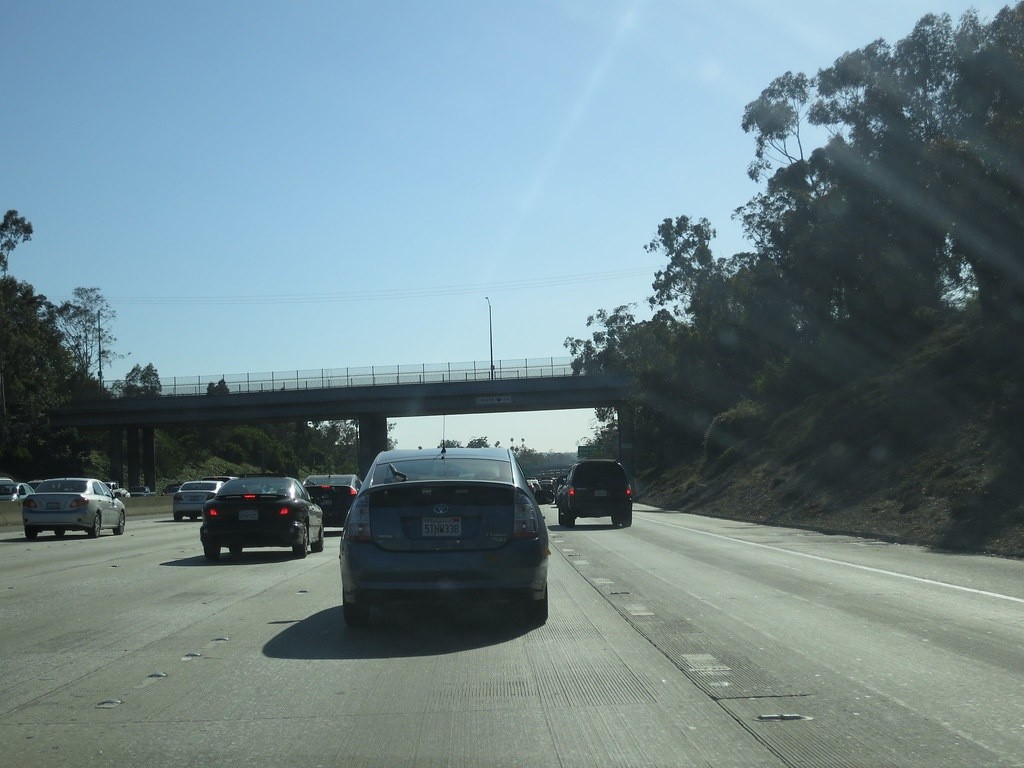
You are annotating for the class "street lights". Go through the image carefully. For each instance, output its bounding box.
[485,296,493,380]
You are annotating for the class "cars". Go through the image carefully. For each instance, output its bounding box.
[303,474,363,528]
[0,474,176,501]
[526,459,632,527]
[200,476,324,560]
[340,441,550,625]
[173,481,226,520]
[23,477,126,540]
